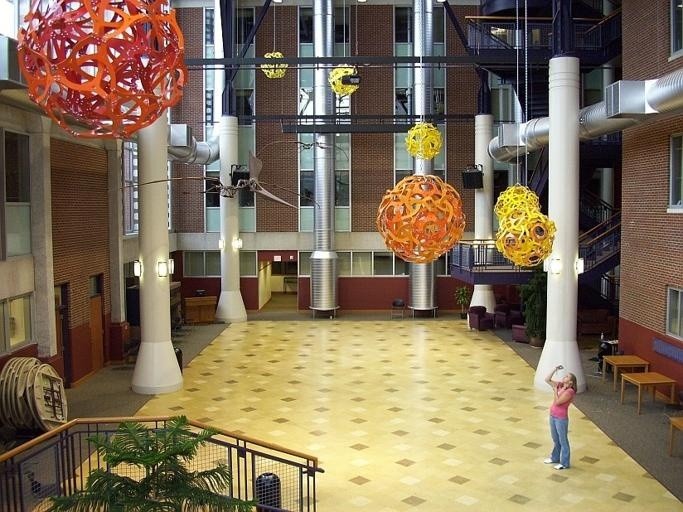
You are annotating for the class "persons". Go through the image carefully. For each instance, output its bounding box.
[544,363,577,469]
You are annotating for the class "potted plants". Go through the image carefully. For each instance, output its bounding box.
[521,272,547,350]
[454,285,472,319]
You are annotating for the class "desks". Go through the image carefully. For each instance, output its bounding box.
[602,354,683,457]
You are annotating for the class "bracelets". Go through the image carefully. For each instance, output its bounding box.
[553,367,556,371]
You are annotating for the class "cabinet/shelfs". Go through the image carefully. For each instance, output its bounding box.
[182,297,218,325]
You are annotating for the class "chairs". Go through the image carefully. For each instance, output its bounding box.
[389,298,406,320]
[468,302,530,343]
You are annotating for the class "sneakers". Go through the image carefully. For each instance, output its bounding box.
[543,458,552,464]
[554,463,565,470]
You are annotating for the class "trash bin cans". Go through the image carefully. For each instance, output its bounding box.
[255,472,282,512]
[598,340,618,373]
[174,348,182,373]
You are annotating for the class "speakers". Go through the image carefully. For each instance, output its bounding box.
[462,171,483,189]
[232,171,251,185]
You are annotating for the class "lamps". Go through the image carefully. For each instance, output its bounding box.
[328,1,362,98]
[493,1,546,218]
[498,1,555,267]
[14,1,188,144]
[406,2,446,161]
[374,172,467,265]
[261,1,289,81]
